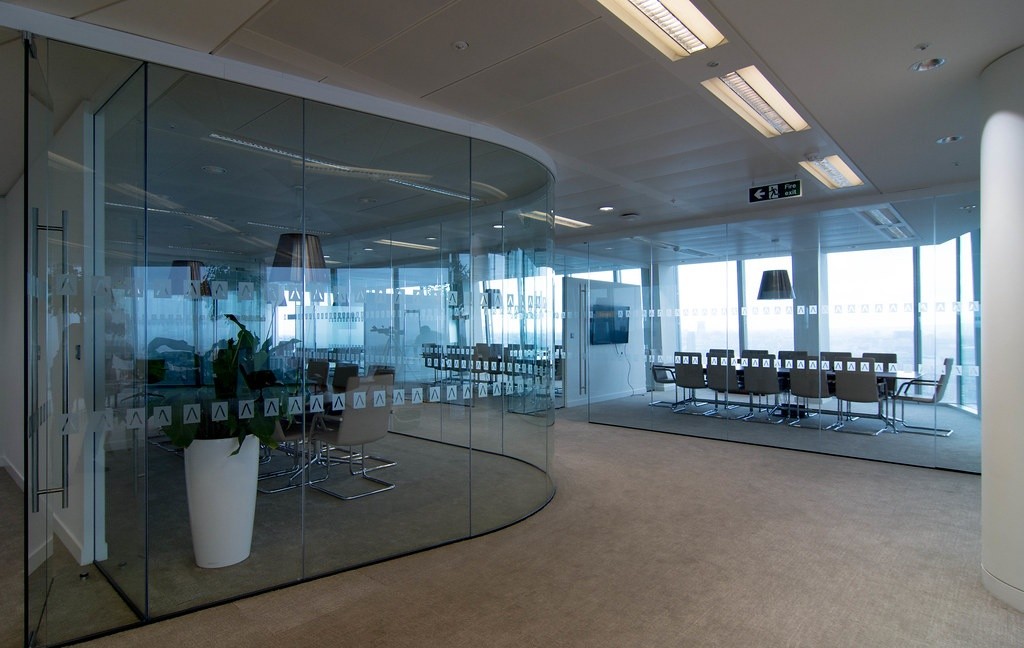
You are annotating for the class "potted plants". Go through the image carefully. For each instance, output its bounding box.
[153,315,299,569]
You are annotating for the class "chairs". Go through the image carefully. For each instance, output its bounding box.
[422,344,564,399]
[649,350,954,438]
[122,357,397,501]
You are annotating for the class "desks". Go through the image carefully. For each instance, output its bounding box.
[652,364,924,434]
[420,352,556,394]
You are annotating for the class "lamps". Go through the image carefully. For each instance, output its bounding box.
[170,225,205,296]
[482,288,502,310]
[757,239,795,301]
[269,186,330,286]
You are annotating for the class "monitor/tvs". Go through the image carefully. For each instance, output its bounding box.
[590,305,630,345]
